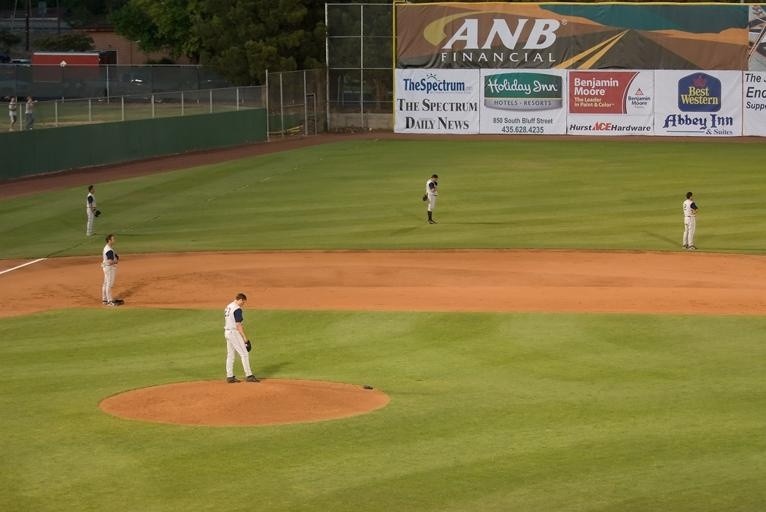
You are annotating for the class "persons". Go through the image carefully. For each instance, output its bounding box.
[25,96,38,129]
[426,175,438,224]
[101,234,118,306]
[683,192,698,250]
[225,292,259,383]
[86,185,97,237]
[8,96,17,128]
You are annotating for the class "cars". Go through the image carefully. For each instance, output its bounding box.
[119,66,184,104]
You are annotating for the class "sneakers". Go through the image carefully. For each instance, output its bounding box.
[246,375,257,382]
[103,300,118,306]
[428,220,436,224]
[227,376,240,383]
[682,244,697,250]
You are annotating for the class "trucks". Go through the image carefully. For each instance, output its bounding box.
[0,50,117,102]
